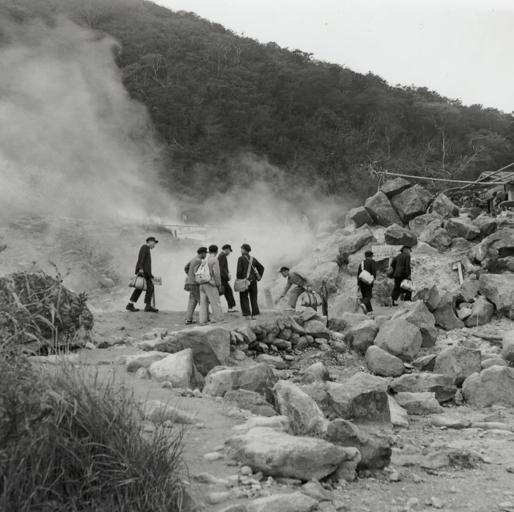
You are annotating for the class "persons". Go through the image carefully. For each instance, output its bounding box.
[126,237,159,313]
[357,250,377,315]
[275,266,313,310]
[217,244,238,313]
[184,246,211,324]
[234,243,265,317]
[386,245,413,307]
[194,245,227,324]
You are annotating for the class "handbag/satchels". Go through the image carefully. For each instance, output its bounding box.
[359,260,374,285]
[234,256,253,292]
[128,272,147,290]
[298,285,322,306]
[244,255,260,280]
[184,282,199,292]
[400,278,415,292]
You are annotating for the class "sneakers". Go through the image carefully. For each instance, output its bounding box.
[360,304,367,315]
[144,306,158,312]
[367,310,375,315]
[185,320,196,325]
[211,318,225,323]
[392,300,399,306]
[228,309,239,312]
[251,315,259,320]
[200,321,209,326]
[246,316,252,320]
[283,307,296,311]
[126,303,140,311]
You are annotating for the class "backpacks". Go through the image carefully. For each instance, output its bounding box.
[195,261,212,285]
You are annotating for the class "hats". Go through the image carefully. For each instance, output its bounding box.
[146,237,158,243]
[365,250,373,255]
[222,244,232,252]
[241,244,251,252]
[403,245,412,252]
[197,247,208,254]
[279,267,289,272]
